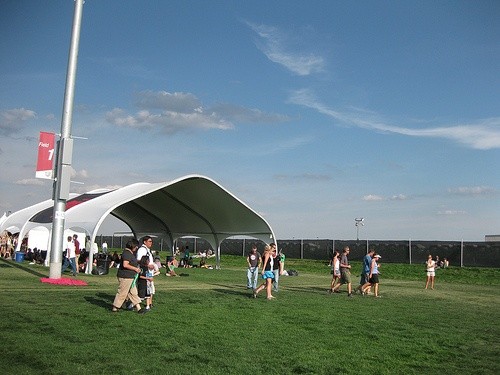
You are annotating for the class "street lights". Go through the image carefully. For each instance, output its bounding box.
[354,217,364,240]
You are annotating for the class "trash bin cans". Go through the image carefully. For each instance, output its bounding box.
[14,252,25,262]
[96,253,109,273]
[180,258,185,267]
[167,256,173,272]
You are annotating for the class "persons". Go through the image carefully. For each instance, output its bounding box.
[127,256,151,310]
[278,249,286,276]
[253,243,277,299]
[94,241,122,275]
[30,250,46,265]
[331,246,353,296]
[200,257,209,269]
[354,249,375,295]
[330,249,342,293]
[198,247,215,257]
[0,230,28,259]
[62,234,98,275]
[153,246,181,278]
[435,256,449,269]
[247,245,261,290]
[183,246,193,268]
[361,253,381,298]
[425,255,436,291]
[111,238,146,314]
[135,235,155,309]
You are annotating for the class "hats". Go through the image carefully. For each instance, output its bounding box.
[373,255,381,259]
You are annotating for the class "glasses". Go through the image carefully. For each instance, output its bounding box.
[346,249,350,252]
[146,240,153,243]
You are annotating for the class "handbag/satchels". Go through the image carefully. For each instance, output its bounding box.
[330,270,339,274]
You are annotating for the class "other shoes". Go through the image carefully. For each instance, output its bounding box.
[374,296,381,298]
[336,291,340,293]
[112,306,119,312]
[331,288,334,293]
[363,289,367,295]
[366,292,373,295]
[146,306,150,312]
[348,292,355,296]
[125,299,133,311]
[358,287,364,297]
[425,286,428,290]
[266,296,276,300]
[430,287,434,290]
[247,284,252,288]
[144,303,155,308]
[137,307,148,314]
[69,272,77,276]
[253,289,258,299]
